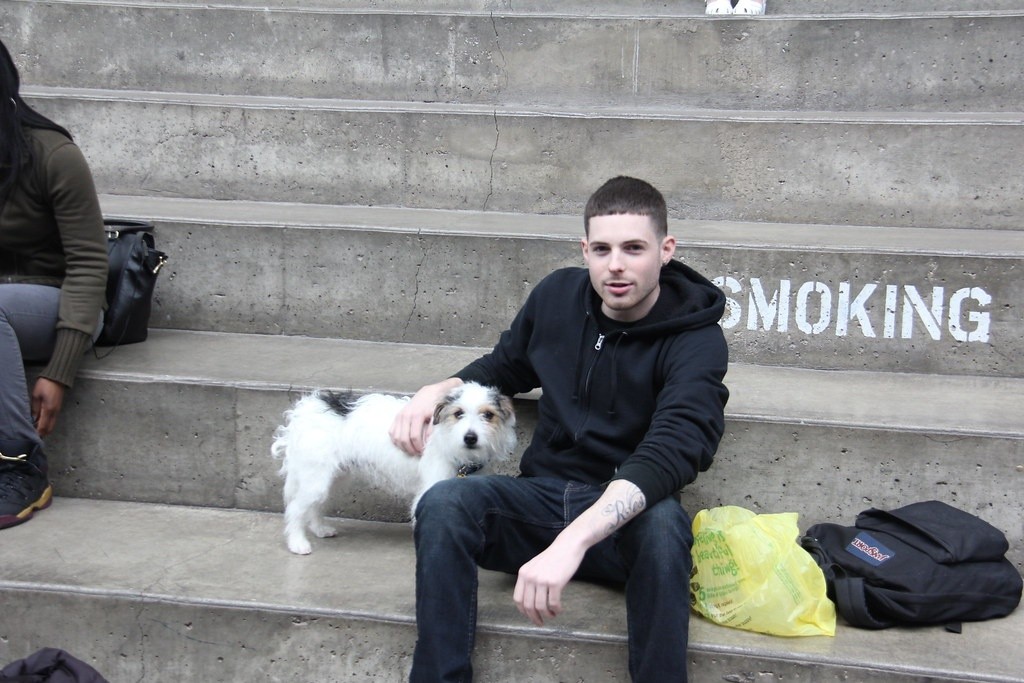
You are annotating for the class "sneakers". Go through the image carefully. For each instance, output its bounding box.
[0,443,52,529]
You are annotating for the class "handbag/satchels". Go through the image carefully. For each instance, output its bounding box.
[687,506,837,637]
[93,219,168,359]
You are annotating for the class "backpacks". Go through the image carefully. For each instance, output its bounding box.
[801,500,1023,634]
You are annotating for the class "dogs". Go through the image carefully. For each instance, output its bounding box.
[270,380,517,554]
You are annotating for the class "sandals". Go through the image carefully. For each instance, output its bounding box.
[733,0,766,16]
[705,0,732,15]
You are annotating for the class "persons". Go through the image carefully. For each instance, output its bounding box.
[0,40,108,530]
[390,176,728,683]
